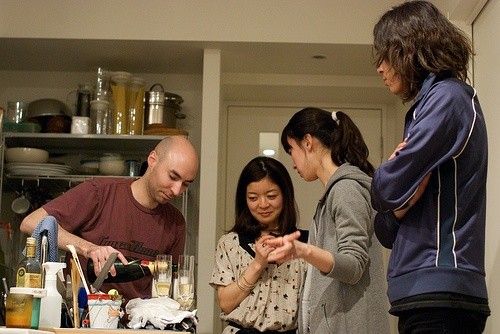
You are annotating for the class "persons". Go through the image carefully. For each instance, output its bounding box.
[20,136,198,330]
[208,156,309,334]
[369,0,491,334]
[265,107,389,334]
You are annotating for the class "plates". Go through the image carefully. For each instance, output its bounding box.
[6,162,73,175]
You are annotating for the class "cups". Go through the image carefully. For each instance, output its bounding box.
[125,160,139,176]
[6,293,33,327]
[30,297,41,330]
[152,255,194,310]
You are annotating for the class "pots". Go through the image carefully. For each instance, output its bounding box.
[146,83,186,128]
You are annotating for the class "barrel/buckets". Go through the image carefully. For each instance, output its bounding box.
[84,294,122,329]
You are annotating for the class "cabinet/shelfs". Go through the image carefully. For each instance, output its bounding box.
[0,132,186,263]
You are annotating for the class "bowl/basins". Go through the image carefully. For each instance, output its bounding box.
[100,156,124,175]
[27,99,72,120]
[6,148,49,163]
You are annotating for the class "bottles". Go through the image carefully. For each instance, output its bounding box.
[90,100,110,135]
[76,81,93,117]
[109,71,145,135]
[87,256,178,284]
[7,100,26,123]
[16,237,43,289]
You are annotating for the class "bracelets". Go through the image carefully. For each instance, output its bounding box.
[237,272,259,293]
